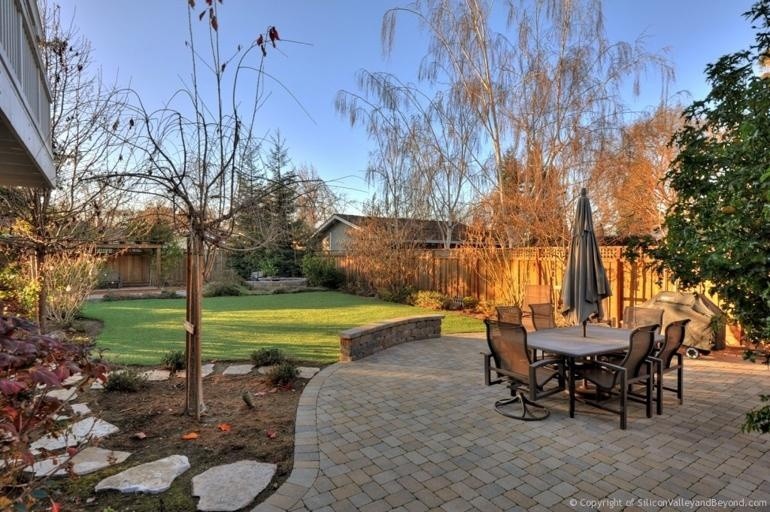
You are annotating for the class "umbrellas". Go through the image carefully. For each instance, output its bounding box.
[561,187,612,335]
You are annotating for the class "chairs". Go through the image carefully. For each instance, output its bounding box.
[597,306,664,394]
[495,306,524,334]
[574,322,661,429]
[483,318,566,420]
[647,318,691,416]
[528,303,557,330]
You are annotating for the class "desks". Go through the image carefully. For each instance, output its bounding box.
[527,326,665,418]
